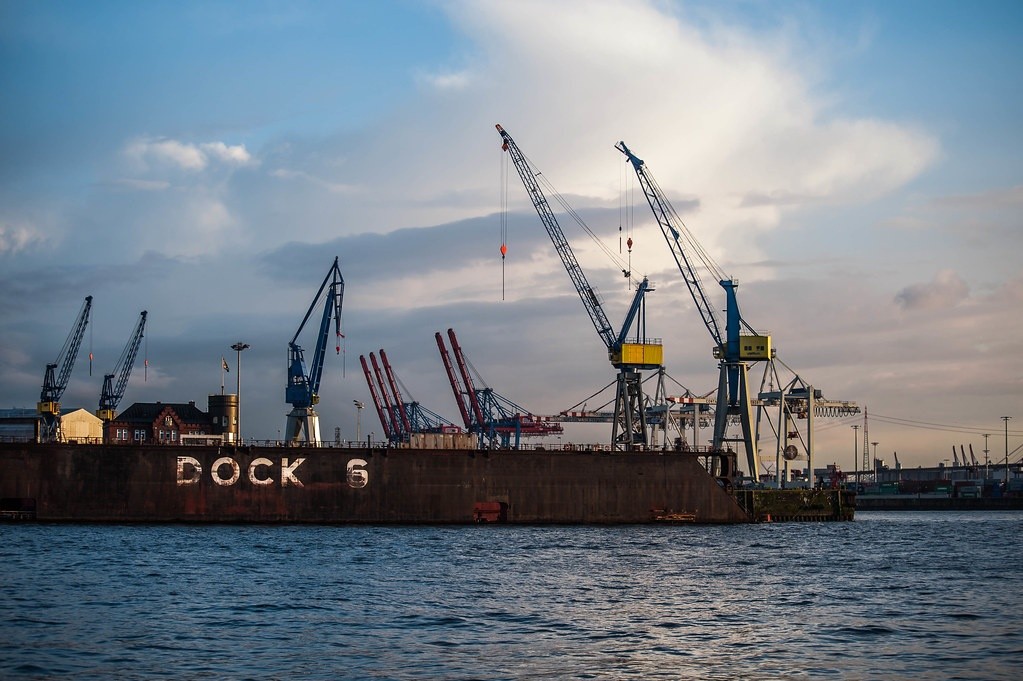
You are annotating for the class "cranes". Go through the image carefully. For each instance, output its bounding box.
[357,121,859,487]
[94,309,150,423]
[36,295,94,425]
[276,254,346,451]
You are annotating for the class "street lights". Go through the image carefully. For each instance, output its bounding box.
[871,442,880,484]
[1001,416,1013,495]
[850,424,862,495]
[231,341,252,447]
[982,433,993,479]
